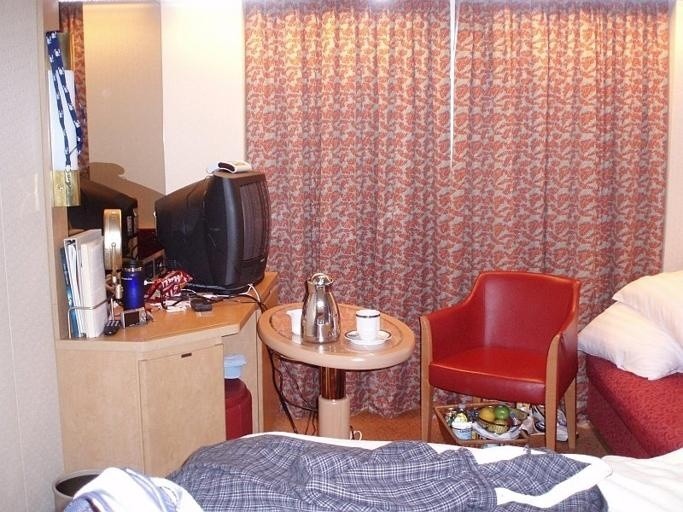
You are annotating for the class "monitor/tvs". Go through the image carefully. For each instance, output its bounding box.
[154,171,271,295]
[67,176,141,271]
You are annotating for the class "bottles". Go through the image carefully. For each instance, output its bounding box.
[120,259,146,310]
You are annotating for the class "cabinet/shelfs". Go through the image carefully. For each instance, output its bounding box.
[57,283,280,479]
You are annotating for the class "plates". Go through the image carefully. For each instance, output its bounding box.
[342,329,393,346]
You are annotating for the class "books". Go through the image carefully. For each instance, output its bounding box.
[58,227,108,338]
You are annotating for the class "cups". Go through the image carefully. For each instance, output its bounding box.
[286,309,305,337]
[355,308,381,341]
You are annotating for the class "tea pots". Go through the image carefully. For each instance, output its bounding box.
[300,271,343,344]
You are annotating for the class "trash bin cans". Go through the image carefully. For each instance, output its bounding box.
[53,469,100,512]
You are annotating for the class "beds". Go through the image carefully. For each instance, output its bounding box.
[163,431,683,511]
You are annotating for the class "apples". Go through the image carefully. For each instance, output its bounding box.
[495,405,510,420]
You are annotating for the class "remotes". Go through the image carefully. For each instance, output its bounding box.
[104,320,121,335]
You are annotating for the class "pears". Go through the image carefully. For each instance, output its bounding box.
[486,420,510,434]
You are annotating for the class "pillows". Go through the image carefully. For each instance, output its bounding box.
[577,271,683,381]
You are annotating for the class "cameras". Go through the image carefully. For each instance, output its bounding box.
[120,307,147,329]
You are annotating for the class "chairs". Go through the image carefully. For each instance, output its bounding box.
[419,270,579,453]
